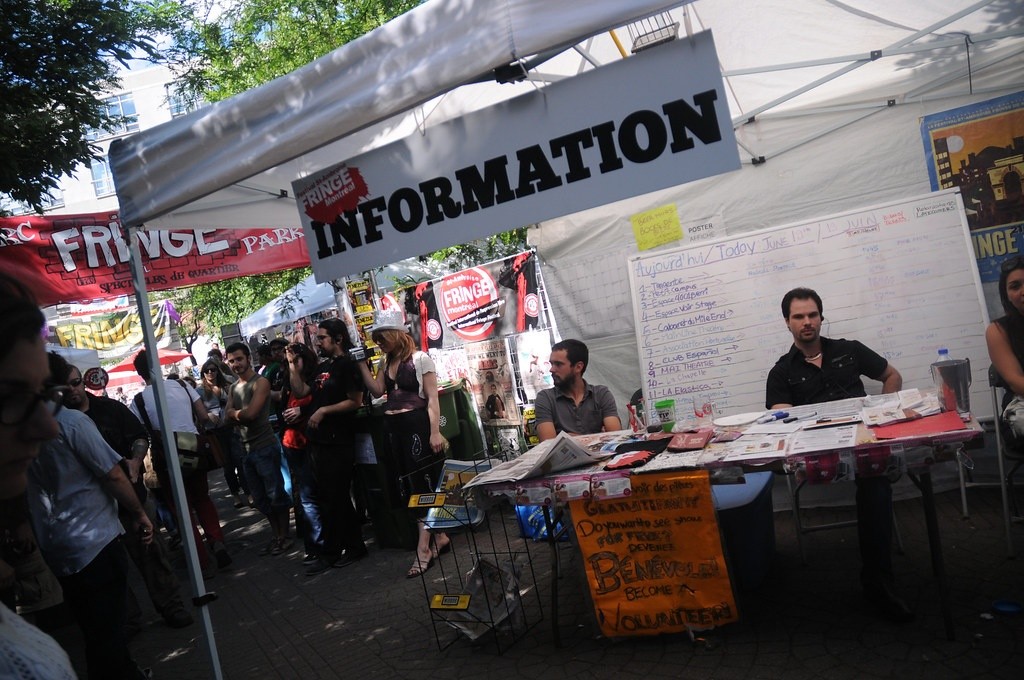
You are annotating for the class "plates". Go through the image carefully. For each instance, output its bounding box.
[712,411,766,426]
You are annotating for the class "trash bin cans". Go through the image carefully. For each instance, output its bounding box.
[437,378,487,461]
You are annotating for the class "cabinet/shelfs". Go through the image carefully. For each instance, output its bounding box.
[399,447,545,657]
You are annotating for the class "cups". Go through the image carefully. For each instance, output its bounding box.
[692,394,714,429]
[654,399,677,433]
[929,359,972,414]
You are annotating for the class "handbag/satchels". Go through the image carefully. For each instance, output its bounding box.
[152,431,203,471]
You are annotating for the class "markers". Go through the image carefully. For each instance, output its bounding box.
[816,417,852,423]
[782,412,817,423]
[757,411,789,424]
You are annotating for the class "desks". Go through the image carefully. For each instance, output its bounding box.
[484,409,986,650]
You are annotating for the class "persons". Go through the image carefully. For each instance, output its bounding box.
[356,309,450,578]
[766,288,912,620]
[195,317,370,576]
[128,350,233,581]
[0,263,195,680]
[535,340,623,625]
[985,254,1024,459]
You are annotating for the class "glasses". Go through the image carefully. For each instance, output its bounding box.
[0,385,70,426]
[68,376,83,386]
[270,348,285,353]
[373,337,385,345]
[203,368,218,374]
[1001,258,1024,272]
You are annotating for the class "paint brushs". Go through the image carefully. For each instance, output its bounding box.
[626,403,647,432]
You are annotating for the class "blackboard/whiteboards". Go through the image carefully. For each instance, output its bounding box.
[626,186,1006,422]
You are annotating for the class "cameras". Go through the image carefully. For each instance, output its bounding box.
[349,346,376,362]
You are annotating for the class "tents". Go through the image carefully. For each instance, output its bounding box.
[0,214,363,350]
[239,257,488,347]
[110,1,1024,680]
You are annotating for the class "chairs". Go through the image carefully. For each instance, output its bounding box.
[988,363,1024,559]
[782,464,905,565]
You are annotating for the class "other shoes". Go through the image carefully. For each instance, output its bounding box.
[269,533,279,549]
[167,535,181,550]
[304,551,320,564]
[248,495,253,504]
[163,610,193,627]
[864,586,912,623]
[233,495,241,507]
[123,620,140,643]
[279,534,295,549]
[213,542,232,569]
[202,566,214,579]
[141,667,153,680]
[304,556,339,574]
[332,548,368,567]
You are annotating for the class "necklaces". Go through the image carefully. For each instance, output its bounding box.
[804,350,822,362]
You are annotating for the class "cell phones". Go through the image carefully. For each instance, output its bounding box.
[292,343,303,354]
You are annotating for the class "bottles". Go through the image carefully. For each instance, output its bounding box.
[935,348,953,363]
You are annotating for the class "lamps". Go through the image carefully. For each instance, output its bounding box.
[630,23,680,54]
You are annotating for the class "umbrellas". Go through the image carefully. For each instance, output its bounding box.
[106,348,193,373]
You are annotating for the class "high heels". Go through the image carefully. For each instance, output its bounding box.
[406,555,434,577]
[431,538,450,559]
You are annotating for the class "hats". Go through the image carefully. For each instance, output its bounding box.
[370,309,409,334]
